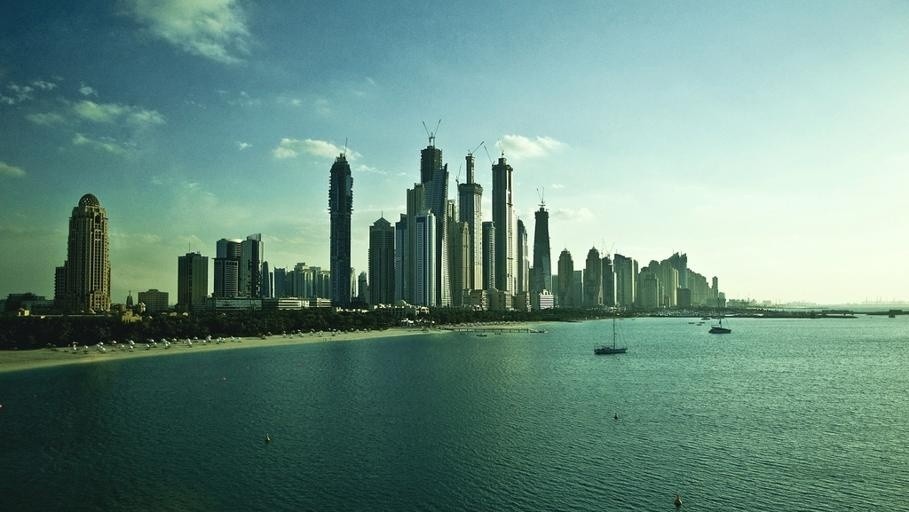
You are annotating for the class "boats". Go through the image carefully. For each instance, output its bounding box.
[476,333,488,338]
[687,317,710,326]
[533,329,548,333]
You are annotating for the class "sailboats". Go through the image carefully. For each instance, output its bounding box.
[593,306,628,355]
[708,296,732,334]
